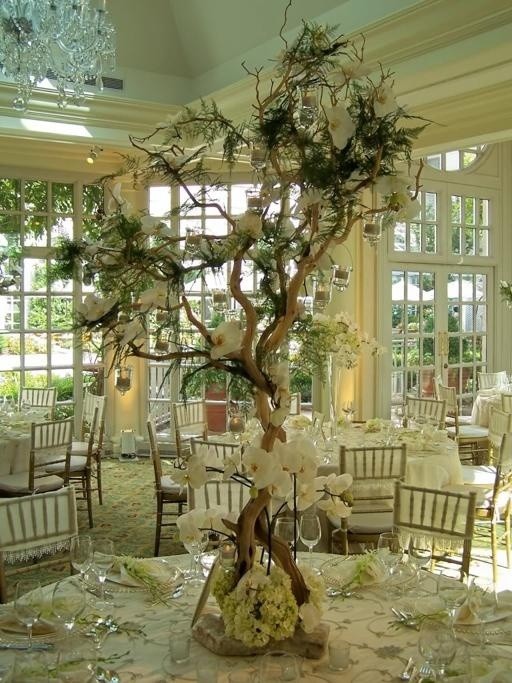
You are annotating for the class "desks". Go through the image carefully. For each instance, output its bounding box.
[0,546,512,682]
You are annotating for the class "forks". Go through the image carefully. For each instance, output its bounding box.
[416,661,433,682]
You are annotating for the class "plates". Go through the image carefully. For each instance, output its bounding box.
[379,560,416,588]
[414,595,510,627]
[194,548,269,570]
[104,557,185,588]
[1,619,57,635]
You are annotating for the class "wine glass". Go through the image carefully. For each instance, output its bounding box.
[202,398,459,469]
[274,517,301,566]
[437,569,470,654]
[300,513,323,576]
[70,535,94,585]
[50,578,87,651]
[13,579,44,654]
[87,538,116,610]
[320,553,371,611]
[466,575,501,656]
[407,533,433,578]
[0,400,37,443]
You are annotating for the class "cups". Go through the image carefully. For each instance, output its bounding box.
[417,613,459,681]
[170,632,191,664]
[326,638,351,672]
[377,532,404,562]
[195,658,220,682]
[260,651,300,681]
[11,650,50,683]
[55,639,97,683]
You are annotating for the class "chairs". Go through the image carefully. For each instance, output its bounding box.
[2,384,109,529]
[143,371,509,586]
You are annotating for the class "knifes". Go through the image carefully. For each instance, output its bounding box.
[69,580,107,601]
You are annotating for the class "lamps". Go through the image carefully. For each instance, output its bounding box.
[1,1,118,118]
